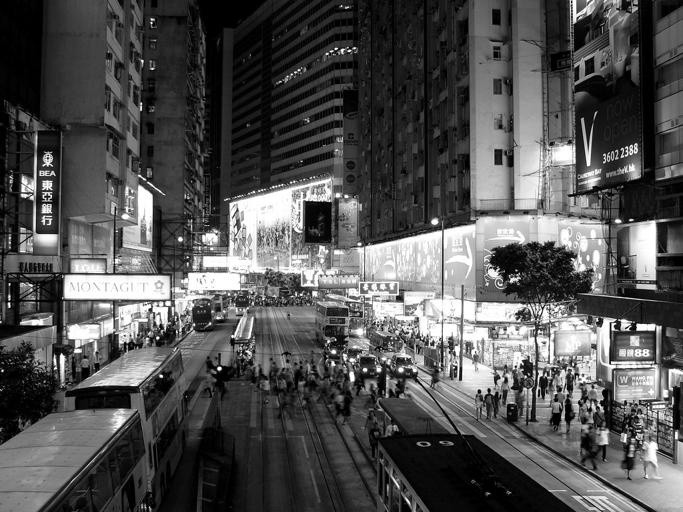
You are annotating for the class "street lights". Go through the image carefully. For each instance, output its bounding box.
[357,239,365,281]
[430,215,444,373]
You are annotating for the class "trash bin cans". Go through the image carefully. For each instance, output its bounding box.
[450,364,458,378]
[507,402,518,422]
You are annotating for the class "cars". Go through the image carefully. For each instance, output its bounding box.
[325,331,418,378]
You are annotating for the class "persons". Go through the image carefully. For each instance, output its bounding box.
[429,367,441,389]
[364,411,377,433]
[70,301,193,383]
[195,351,409,424]
[204,288,319,310]
[349,314,462,379]
[384,419,399,438]
[368,425,381,462]
[472,354,666,481]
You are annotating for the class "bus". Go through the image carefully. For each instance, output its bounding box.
[316,293,364,349]
[0,347,188,512]
[378,378,576,512]
[192,290,250,332]
